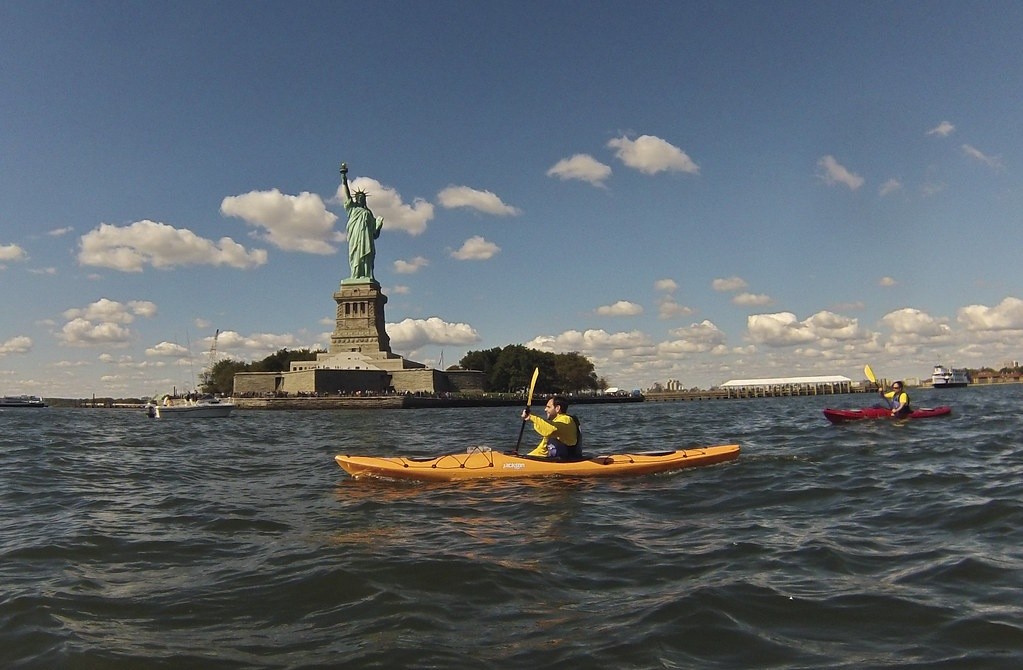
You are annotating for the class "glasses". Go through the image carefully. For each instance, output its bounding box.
[893,387,899,389]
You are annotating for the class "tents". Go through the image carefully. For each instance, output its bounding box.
[606,387,621,393]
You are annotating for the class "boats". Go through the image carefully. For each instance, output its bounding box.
[821,403,953,423]
[335,442,741,478]
[931,363,971,389]
[153,393,237,418]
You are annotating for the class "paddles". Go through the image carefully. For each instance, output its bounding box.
[863,364,894,409]
[513,366,541,456]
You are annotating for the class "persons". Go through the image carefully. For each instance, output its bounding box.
[521,394,581,460]
[163,397,173,407]
[514,388,632,397]
[339,162,384,280]
[879,381,910,415]
[222,390,452,398]
